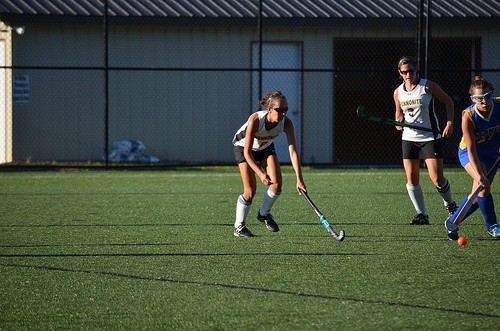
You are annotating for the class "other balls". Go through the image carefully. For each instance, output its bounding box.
[457,235,468,246]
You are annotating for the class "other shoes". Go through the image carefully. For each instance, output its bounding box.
[445,217,459,241]
[487,223,500,239]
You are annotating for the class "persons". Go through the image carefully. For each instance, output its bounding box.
[232,91,308,238]
[393,57,459,225]
[445,77,500,241]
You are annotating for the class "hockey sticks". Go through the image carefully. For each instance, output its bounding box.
[445,154,500,232]
[357,106,444,134]
[301,189,345,241]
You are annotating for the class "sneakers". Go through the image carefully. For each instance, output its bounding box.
[257,210,279,232]
[234,224,253,237]
[409,213,430,225]
[446,201,459,216]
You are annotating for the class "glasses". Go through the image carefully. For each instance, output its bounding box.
[273,107,288,112]
[400,68,415,75]
[473,91,493,103]
